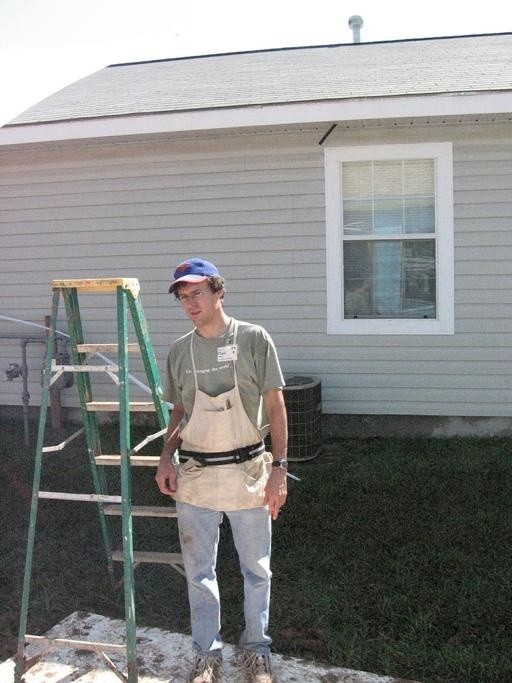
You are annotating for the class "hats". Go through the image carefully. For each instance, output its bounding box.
[167,258,221,293]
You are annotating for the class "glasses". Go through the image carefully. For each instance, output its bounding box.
[175,289,211,303]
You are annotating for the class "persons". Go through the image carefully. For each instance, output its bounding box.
[150,257,290,683]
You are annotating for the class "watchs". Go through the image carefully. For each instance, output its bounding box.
[269,459,287,471]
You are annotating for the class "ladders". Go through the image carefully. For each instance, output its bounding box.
[16,278,186,683]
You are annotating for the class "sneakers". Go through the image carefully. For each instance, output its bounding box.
[190,651,223,682]
[243,650,274,683]
